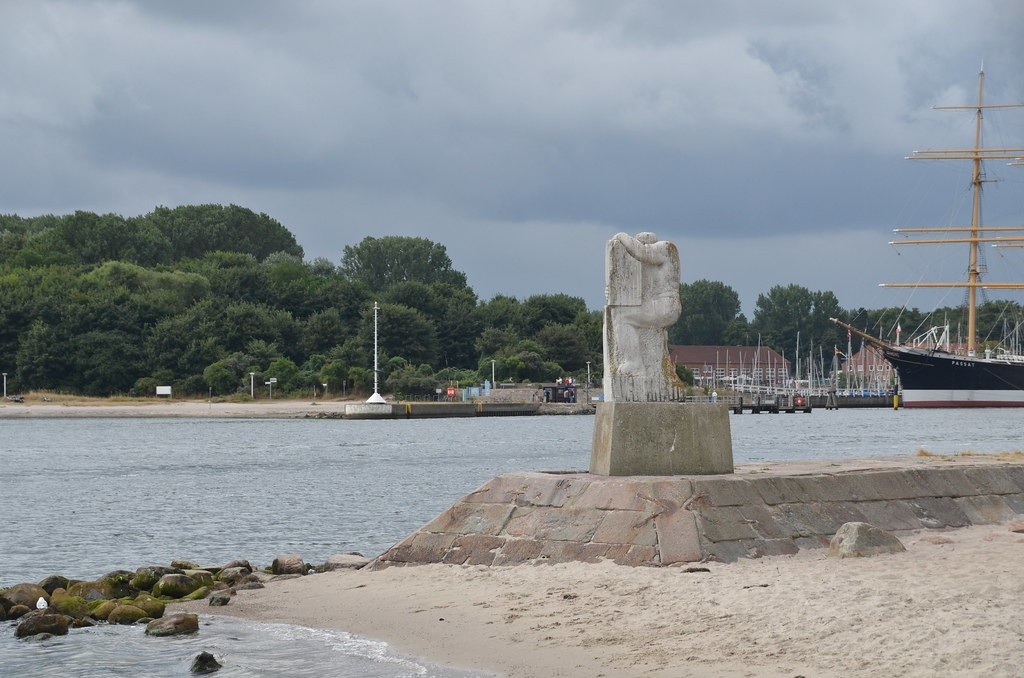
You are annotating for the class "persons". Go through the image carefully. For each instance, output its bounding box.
[556,376,576,386]
[602,231,682,377]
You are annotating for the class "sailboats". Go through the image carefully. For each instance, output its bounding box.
[827,57,1024,413]
